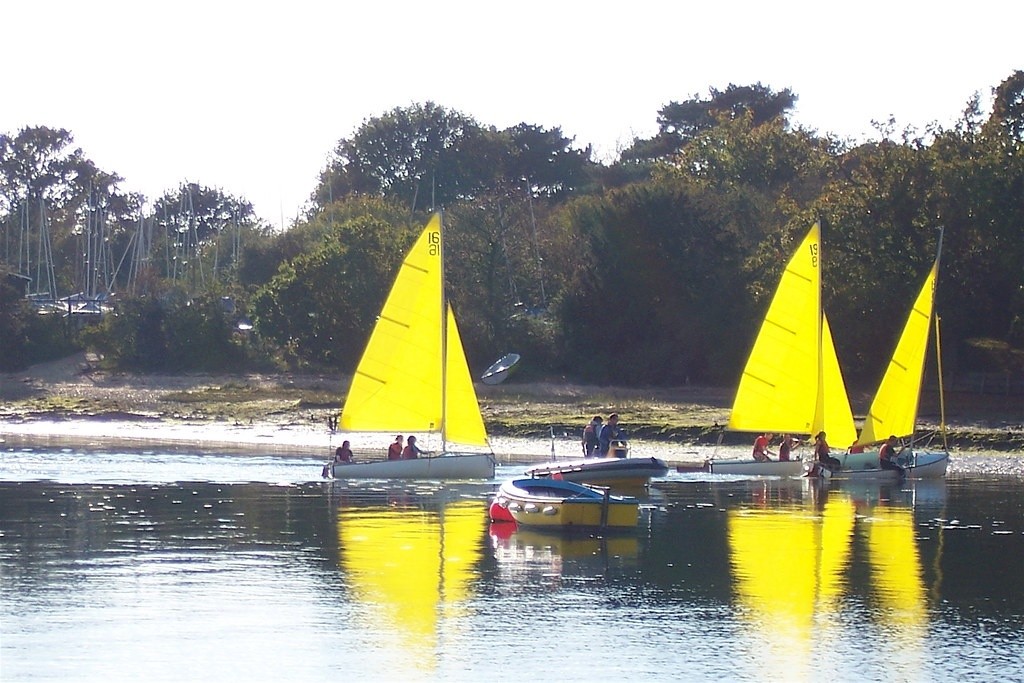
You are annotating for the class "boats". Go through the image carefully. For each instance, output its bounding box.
[490,467,641,535]
[530,456,669,489]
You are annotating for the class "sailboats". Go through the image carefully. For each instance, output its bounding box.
[320,204,499,482]
[700,212,950,482]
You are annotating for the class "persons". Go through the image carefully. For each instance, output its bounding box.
[583,413,627,458]
[753,430,906,479]
[334,433,436,464]
